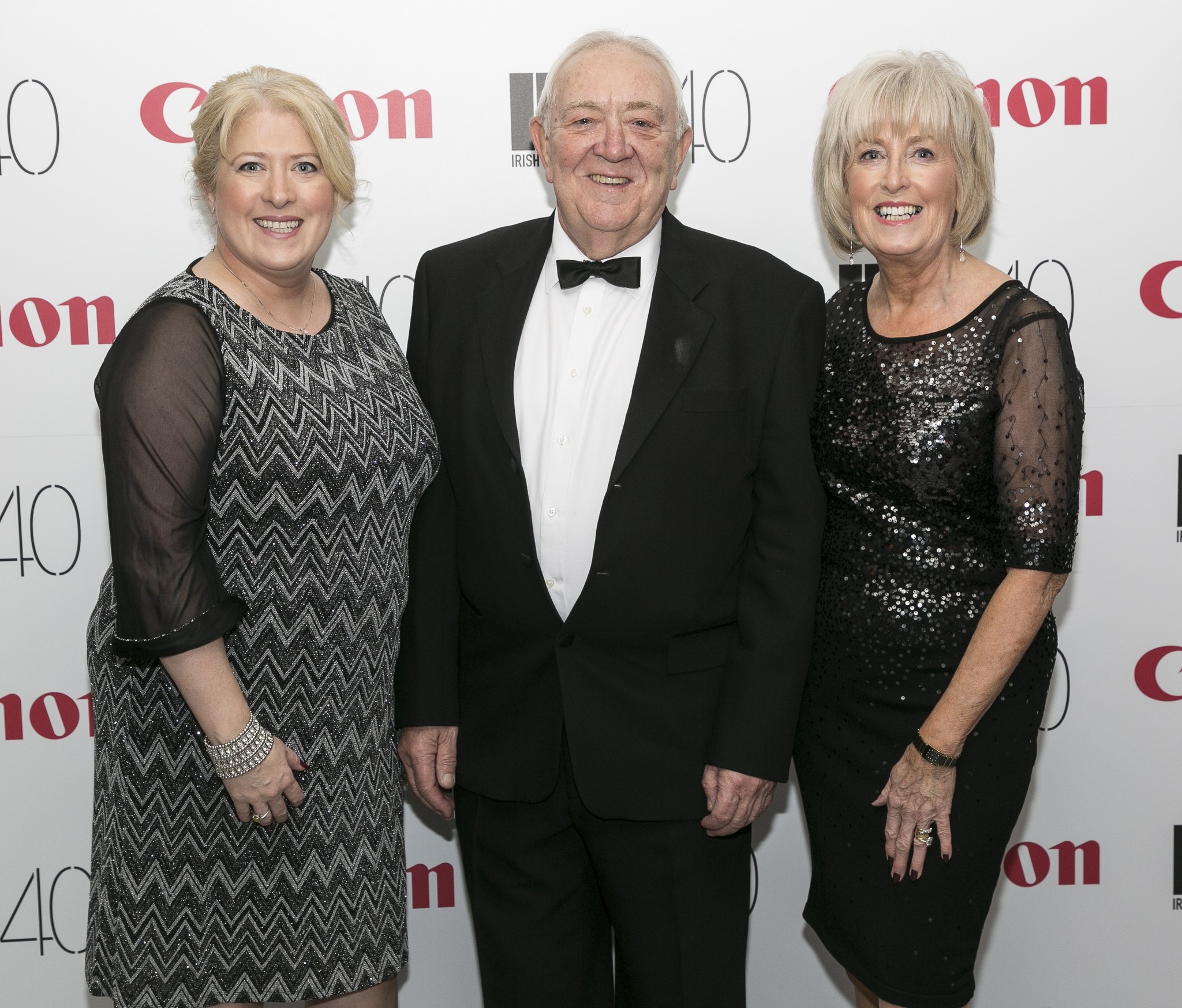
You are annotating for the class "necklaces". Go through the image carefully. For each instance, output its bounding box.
[209,245,314,338]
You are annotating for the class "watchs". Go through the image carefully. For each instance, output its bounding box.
[913,728,963,767]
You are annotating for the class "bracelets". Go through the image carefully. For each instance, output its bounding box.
[203,709,275,780]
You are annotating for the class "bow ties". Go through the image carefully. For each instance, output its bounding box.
[556,256,641,289]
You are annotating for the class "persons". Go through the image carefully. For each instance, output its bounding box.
[82,62,446,1008]
[402,34,823,1008]
[789,48,1088,1008]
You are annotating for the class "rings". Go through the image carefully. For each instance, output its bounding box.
[915,826,935,846]
[252,807,270,824]
